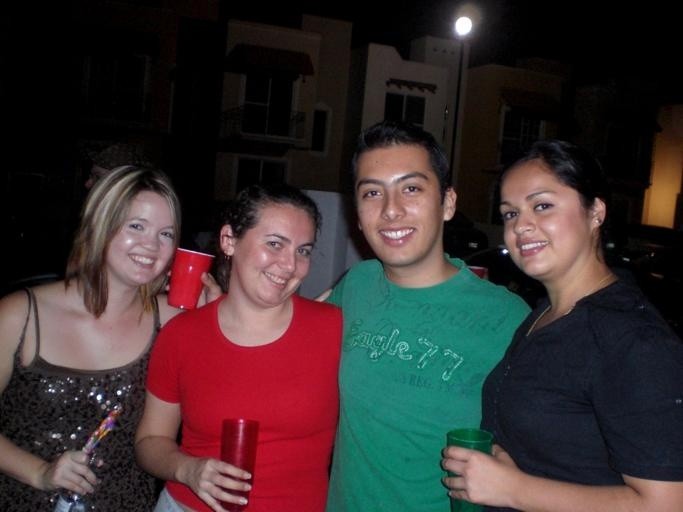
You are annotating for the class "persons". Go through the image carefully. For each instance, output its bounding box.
[0,164,223,511]
[313,139,683,511]
[133,182,343,512]
[322,119,532,512]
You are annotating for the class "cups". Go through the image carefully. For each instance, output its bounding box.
[167,247,215,312]
[220,419,260,512]
[447,428,493,512]
[468,265,485,279]
